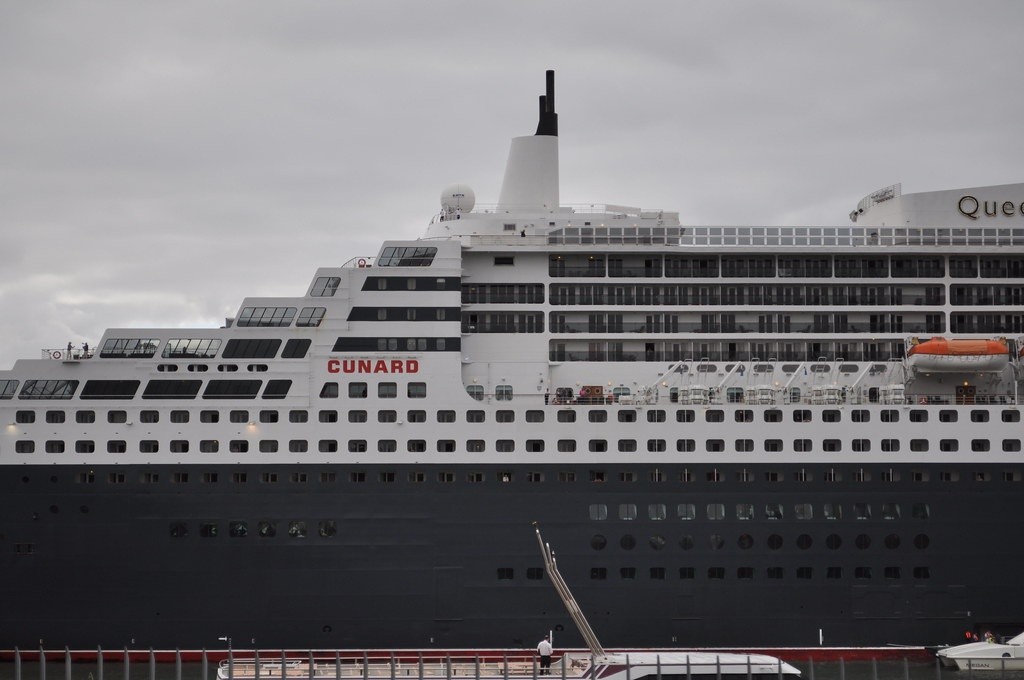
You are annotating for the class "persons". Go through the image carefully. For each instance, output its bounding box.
[537,634,553,675]
[82,342,88,360]
[964,629,971,643]
[521,229,525,237]
[440,209,445,222]
[544,388,550,404]
[456,208,462,219]
[995,632,1001,643]
[972,632,978,642]
[67,342,75,359]
[984,630,992,641]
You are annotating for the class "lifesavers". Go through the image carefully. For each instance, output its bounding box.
[358,259,366,266]
[53,351,61,359]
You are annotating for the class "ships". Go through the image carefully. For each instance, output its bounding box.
[1,66,1023,678]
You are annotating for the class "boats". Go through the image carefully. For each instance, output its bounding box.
[907,340,1007,372]
[879,383,906,403]
[812,384,842,403]
[746,386,776,405]
[678,384,710,405]
[618,395,646,404]
[215,518,802,680]
[939,631,1023,670]
